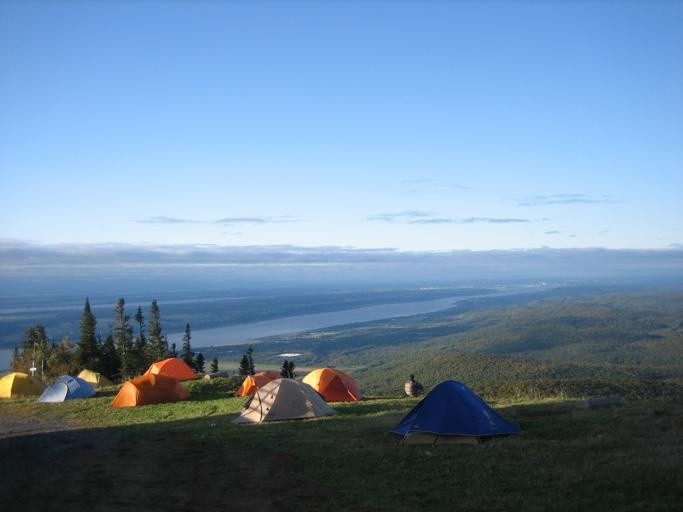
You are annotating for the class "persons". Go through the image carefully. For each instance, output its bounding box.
[404,373,423,397]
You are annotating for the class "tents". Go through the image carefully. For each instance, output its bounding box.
[228,371,283,398]
[142,357,197,382]
[301,366,364,401]
[391,378,523,437]
[110,373,190,408]
[35,374,95,403]
[231,377,340,424]
[0,372,47,398]
[76,369,113,390]
